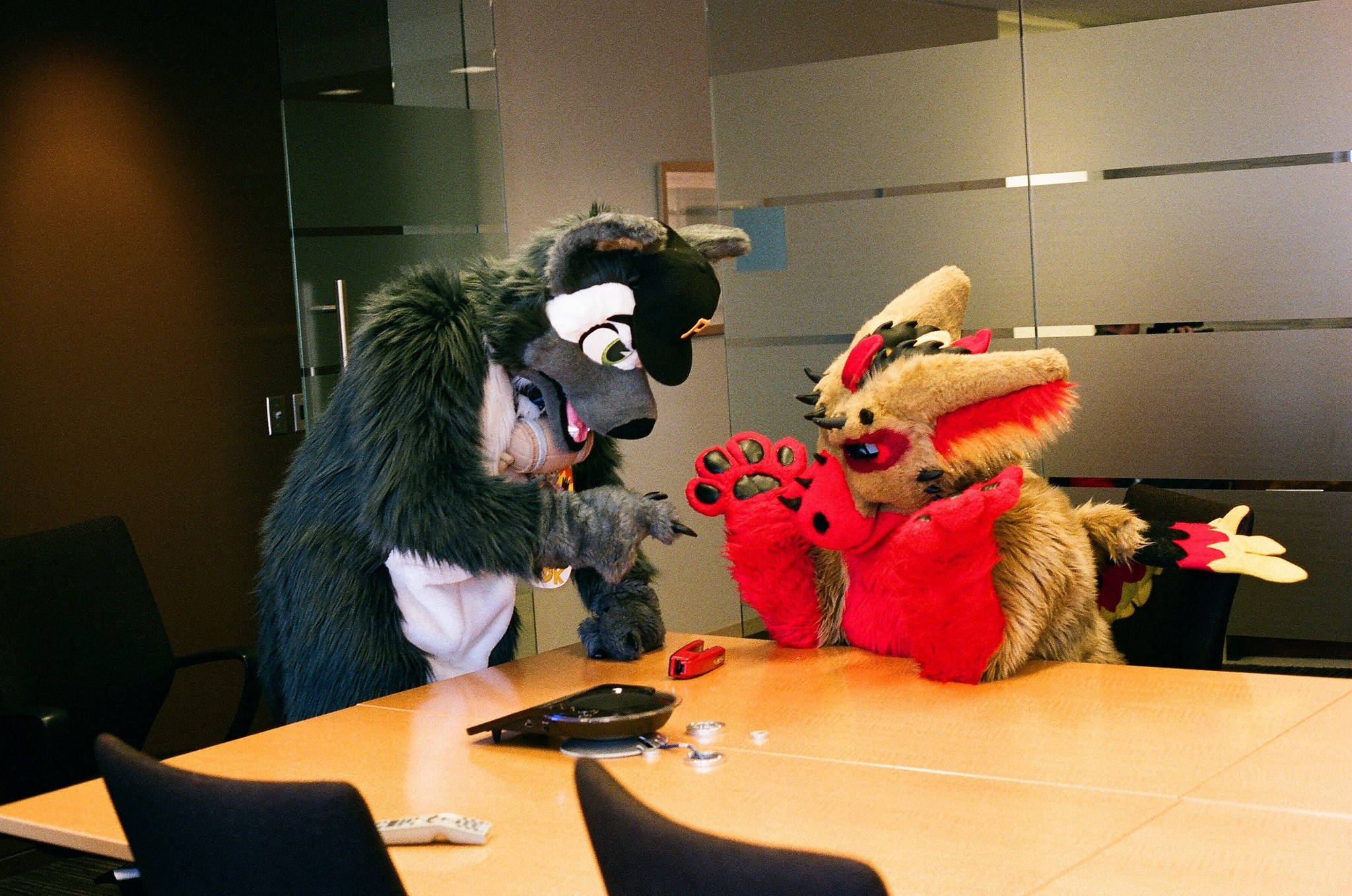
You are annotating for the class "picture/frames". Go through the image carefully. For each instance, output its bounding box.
[656,160,724,339]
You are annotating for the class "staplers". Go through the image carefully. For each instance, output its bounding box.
[668,639,725,680]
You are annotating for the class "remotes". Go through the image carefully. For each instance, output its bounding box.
[377,813,491,845]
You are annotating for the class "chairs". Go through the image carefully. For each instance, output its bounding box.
[1109,483,1253,672]
[94,732,407,896]
[574,757,889,896]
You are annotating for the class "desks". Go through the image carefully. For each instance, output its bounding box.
[0,632,1352,896]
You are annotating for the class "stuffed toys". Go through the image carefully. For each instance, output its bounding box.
[685,260,1308,684]
[258,202,750,719]
